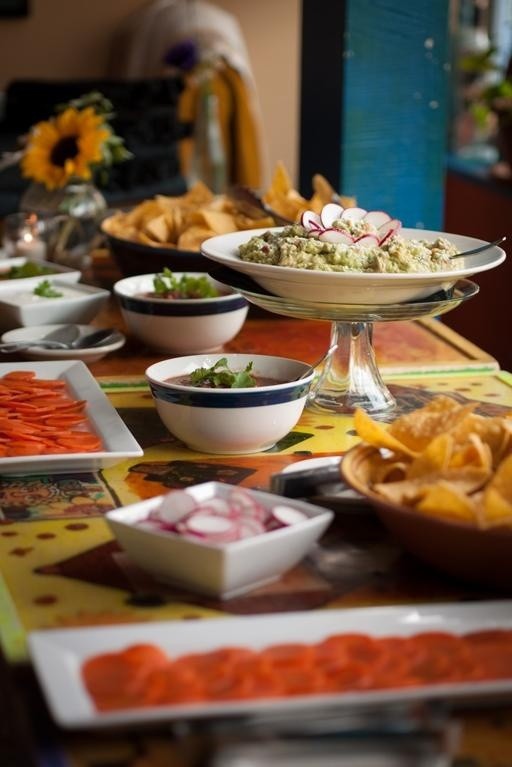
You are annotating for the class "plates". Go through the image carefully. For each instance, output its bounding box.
[0,323,144,477]
[26,602,511,726]
[0,257,110,328]
[105,455,366,601]
[201,226,507,303]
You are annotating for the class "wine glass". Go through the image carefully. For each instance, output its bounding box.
[207,265,480,416]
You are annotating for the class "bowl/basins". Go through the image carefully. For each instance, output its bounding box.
[102,212,274,315]
[341,441,512,571]
[113,271,315,454]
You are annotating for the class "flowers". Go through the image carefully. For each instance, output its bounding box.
[20,92,135,192]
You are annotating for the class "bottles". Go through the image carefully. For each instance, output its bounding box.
[2,212,47,260]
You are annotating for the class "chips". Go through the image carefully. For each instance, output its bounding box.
[114,181,276,252]
[265,163,357,223]
[353,396,512,530]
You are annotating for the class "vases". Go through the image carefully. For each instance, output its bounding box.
[19,184,108,271]
